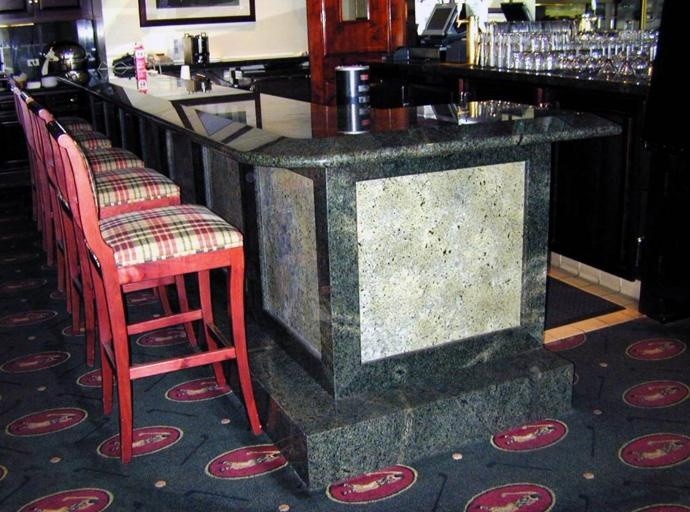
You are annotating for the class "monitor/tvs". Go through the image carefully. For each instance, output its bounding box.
[421,2,457,36]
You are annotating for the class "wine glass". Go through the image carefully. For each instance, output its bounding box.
[556,32,653,79]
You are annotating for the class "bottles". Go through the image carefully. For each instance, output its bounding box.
[536,88,544,118]
[457,79,466,120]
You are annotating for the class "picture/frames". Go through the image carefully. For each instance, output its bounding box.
[139,0,255,27]
